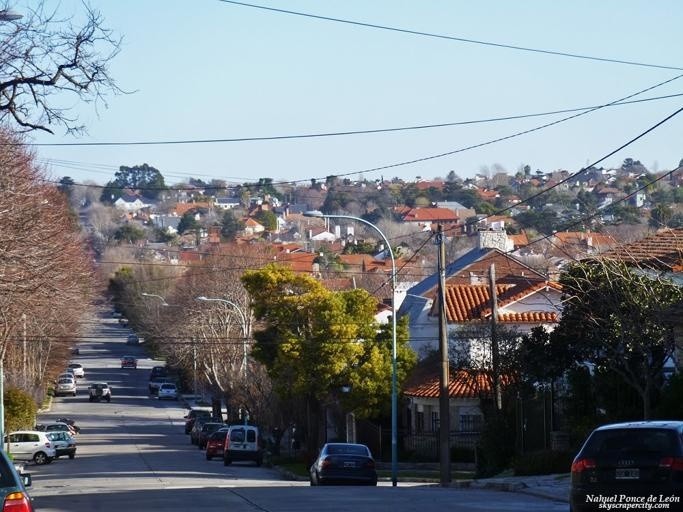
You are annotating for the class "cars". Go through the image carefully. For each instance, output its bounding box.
[1,447,34,512]
[184,409,263,466]
[310,442,378,487]
[56,363,85,397]
[126,334,140,347]
[119,355,138,369]
[0,421,77,466]
[570,420,682,510]
[87,384,113,403]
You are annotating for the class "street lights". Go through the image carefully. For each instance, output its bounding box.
[140,293,248,426]
[302,210,398,486]
